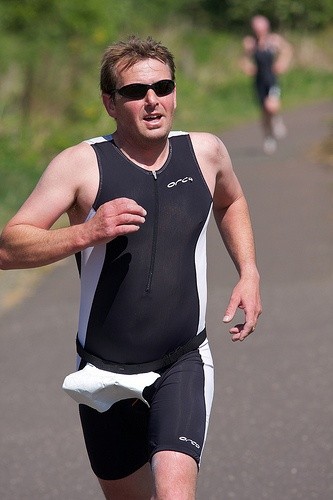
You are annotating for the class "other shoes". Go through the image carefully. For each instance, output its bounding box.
[262,138,276,155]
[269,116,285,140]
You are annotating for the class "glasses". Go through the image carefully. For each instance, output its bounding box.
[114,79,174,100]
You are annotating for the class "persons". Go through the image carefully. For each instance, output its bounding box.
[1,33,263,499]
[238,14,294,154]
